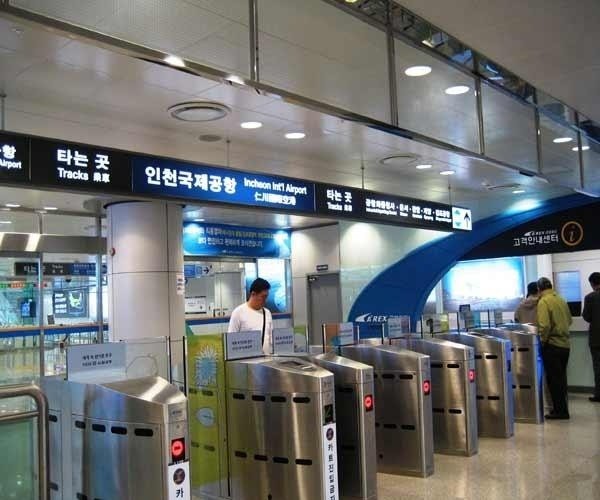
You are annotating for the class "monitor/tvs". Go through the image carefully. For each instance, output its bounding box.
[21,302,36,317]
[568,301,581,316]
[52,287,89,318]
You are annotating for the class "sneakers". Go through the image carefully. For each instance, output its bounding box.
[545,394,600,421]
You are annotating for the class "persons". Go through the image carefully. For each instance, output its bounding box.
[582,272,600,402]
[513,281,540,328]
[537,277,574,419]
[229,278,275,354]
